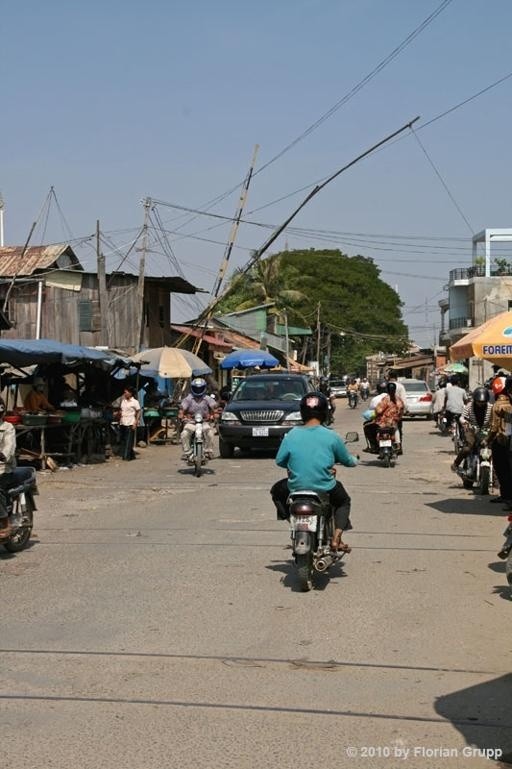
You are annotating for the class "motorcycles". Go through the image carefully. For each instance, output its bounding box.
[181,413,216,477]
[1,466,41,553]
[376,422,404,468]
[360,386,369,402]
[281,432,359,590]
[451,444,489,498]
[347,389,359,409]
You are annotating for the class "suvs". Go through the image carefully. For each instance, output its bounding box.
[216,373,317,458]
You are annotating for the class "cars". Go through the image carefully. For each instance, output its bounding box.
[397,380,433,421]
[328,381,346,397]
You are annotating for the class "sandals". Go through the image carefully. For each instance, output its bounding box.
[330,539,352,553]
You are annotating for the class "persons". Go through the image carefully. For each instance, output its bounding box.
[119,378,221,462]
[314,375,408,460]
[429,363,512,512]
[0,398,18,539]
[24,377,54,411]
[270,392,353,554]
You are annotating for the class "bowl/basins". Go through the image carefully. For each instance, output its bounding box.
[4,414,22,424]
[63,412,80,421]
[49,415,62,422]
[23,415,49,426]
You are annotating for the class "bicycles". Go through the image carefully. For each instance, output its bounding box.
[447,413,461,442]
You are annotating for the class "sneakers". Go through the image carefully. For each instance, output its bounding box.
[489,496,506,503]
[180,452,192,460]
[502,504,512,512]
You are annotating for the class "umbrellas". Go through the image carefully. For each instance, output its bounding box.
[448,307,512,373]
[219,348,279,371]
[127,345,214,438]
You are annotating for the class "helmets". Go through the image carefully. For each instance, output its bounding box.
[190,378,208,397]
[0,398,7,418]
[492,376,508,396]
[473,387,491,409]
[376,380,389,394]
[299,391,329,423]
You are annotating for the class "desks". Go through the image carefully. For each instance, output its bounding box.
[14,419,106,469]
